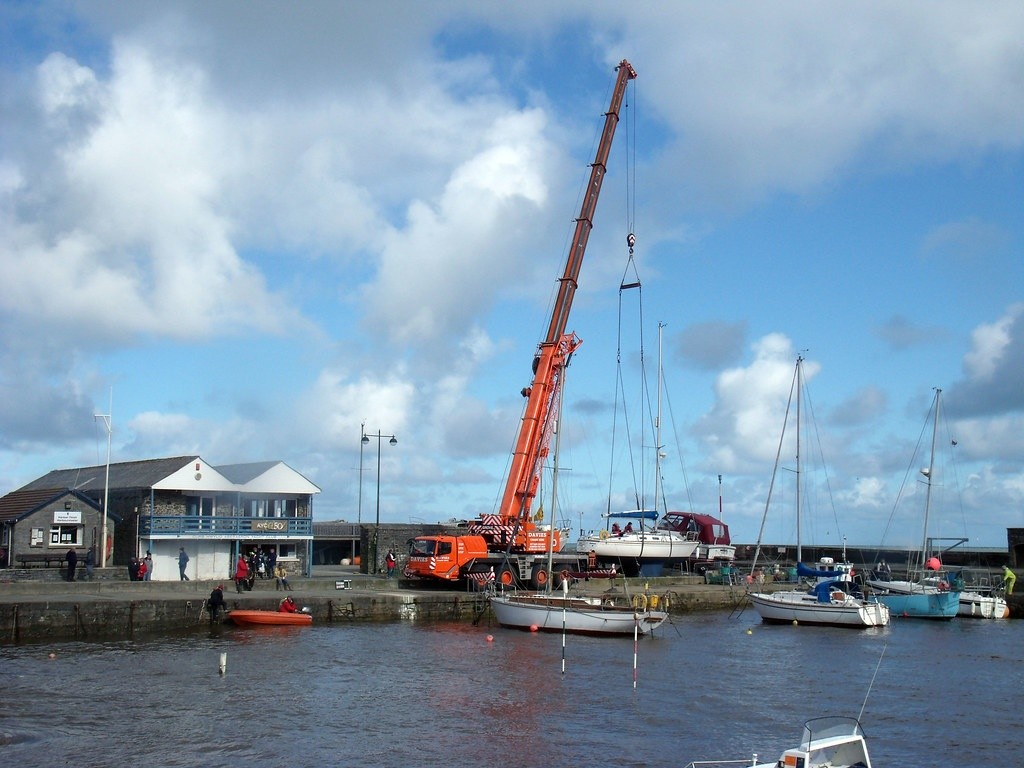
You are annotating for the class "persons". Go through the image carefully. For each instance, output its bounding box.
[66,548,77,582]
[877,559,891,573]
[86,547,94,576]
[237,548,293,594]
[210,586,230,623]
[612,522,633,536]
[386,550,397,578]
[129,553,153,581]
[178,548,189,581]
[280,596,296,613]
[1002,565,1016,595]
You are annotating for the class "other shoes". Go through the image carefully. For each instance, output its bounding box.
[238,591,244,594]
[224,610,232,614]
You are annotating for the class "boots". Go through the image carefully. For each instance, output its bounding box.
[279,585,283,591]
[285,585,289,591]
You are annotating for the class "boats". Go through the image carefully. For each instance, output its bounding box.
[804,386,960,616]
[577,509,736,564]
[487,364,669,634]
[228,610,312,626]
[685,716,872,768]
[746,354,889,625]
[866,569,1007,619]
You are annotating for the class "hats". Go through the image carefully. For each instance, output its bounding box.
[218,585,224,591]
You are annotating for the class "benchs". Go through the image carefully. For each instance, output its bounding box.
[16,553,89,568]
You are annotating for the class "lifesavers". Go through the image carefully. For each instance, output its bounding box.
[599,530,608,540]
[633,593,647,608]
[650,595,659,606]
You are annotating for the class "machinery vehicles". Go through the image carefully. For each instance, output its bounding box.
[406,58,642,592]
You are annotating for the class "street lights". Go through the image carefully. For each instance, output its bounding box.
[361,433,398,524]
